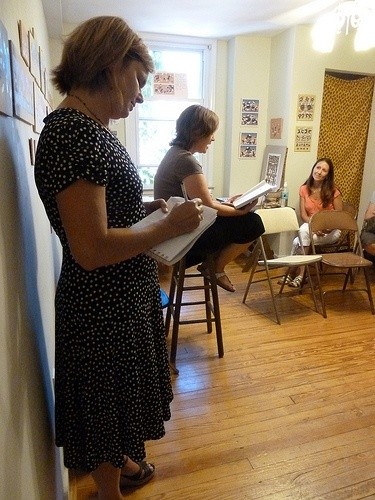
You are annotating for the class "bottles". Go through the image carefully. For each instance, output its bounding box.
[279,182,289,208]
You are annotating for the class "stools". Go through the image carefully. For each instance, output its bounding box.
[163,249,224,363]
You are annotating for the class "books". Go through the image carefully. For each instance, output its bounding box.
[231,178,271,210]
[129,194,219,267]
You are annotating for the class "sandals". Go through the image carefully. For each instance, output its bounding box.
[278,274,311,288]
[196,265,236,293]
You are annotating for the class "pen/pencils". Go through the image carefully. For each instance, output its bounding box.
[181,182,188,201]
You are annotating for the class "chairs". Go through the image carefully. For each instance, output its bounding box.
[299,209,375,320]
[300,202,360,289]
[243,208,323,325]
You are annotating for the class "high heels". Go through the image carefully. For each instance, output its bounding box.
[116,454,154,489]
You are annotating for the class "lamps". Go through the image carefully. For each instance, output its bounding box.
[308,0,375,54]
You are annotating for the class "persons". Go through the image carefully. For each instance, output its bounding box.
[276,157,344,289]
[153,105,266,294]
[359,189,375,257]
[35,15,205,500]
